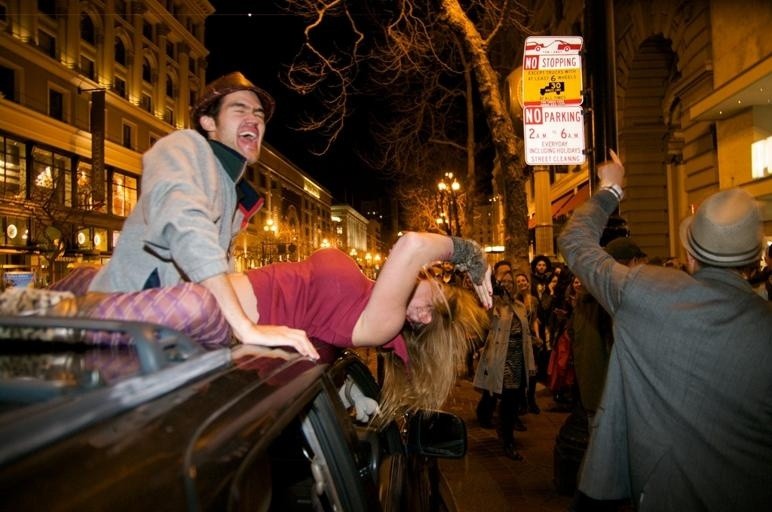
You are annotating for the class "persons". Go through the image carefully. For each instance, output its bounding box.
[1,228,494,418]
[664,246,772,302]
[231,343,303,362]
[83,62,320,360]
[467,216,649,512]
[557,150,771,511]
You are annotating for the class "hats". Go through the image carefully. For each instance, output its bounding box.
[190,71,276,126]
[679,189,762,268]
[605,237,647,259]
[531,256,551,273]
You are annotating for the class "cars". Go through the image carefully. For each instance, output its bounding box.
[0,313,468,512]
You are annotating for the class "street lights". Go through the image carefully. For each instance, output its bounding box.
[435,172,461,230]
[263,219,279,263]
[319,239,381,269]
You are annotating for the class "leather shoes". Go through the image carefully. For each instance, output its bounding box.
[476,400,540,460]
